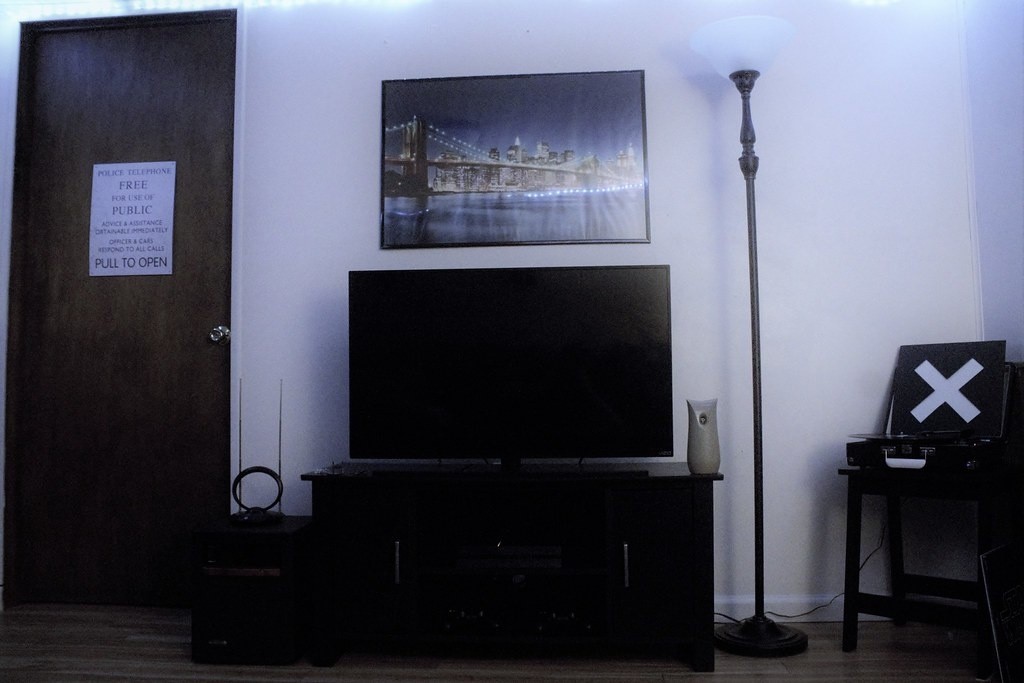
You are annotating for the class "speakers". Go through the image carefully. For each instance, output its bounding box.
[687,398,721,474]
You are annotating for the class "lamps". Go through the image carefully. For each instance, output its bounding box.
[693,14,808,661]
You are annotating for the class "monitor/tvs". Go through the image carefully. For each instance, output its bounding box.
[349,264,675,478]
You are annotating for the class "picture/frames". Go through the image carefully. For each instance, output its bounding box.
[381,70,650,249]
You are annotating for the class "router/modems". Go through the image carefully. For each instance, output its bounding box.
[224,378,289,529]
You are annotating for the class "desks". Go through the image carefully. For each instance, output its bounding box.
[302,461,724,671]
[837,465,1023,679]
